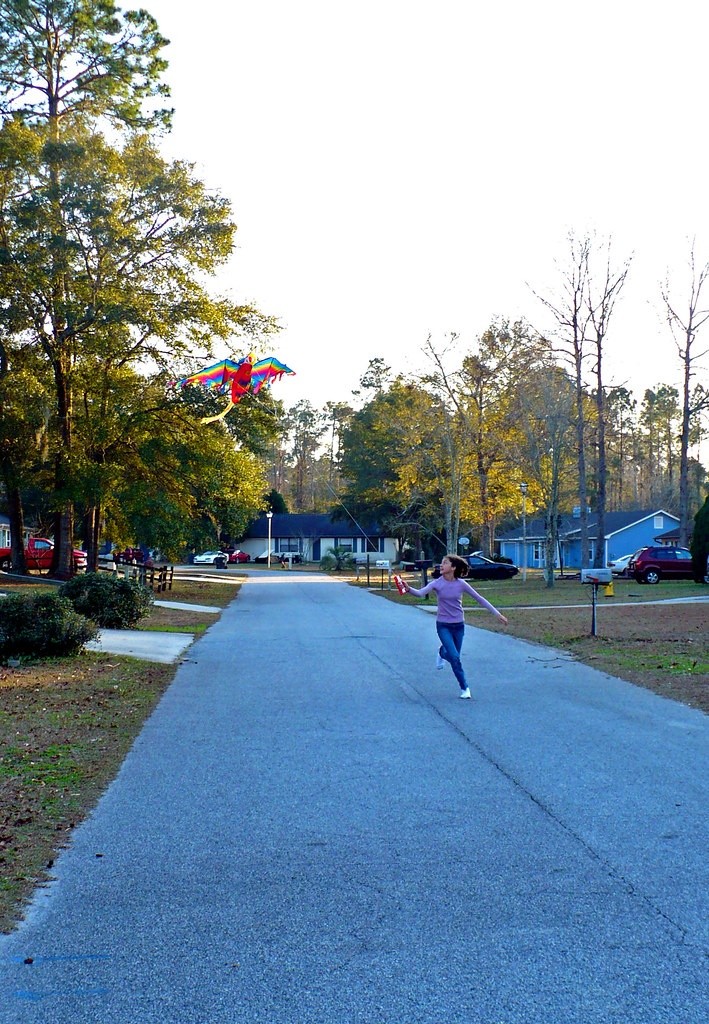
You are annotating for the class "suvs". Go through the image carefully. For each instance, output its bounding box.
[627,546,692,584]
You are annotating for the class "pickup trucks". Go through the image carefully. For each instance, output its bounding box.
[0,538,87,574]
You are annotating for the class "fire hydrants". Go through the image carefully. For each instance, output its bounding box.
[601,578,614,597]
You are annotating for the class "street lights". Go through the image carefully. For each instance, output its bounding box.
[519,482,530,581]
[266,509,273,568]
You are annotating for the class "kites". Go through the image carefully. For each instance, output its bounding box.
[167,352,297,424]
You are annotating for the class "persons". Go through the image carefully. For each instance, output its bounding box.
[394,555,507,699]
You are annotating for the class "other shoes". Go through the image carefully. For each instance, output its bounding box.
[460,687,471,698]
[437,645,445,669]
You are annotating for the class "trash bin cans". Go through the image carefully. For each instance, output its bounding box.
[216,556,228,569]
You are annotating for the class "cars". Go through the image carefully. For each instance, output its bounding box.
[432,555,518,581]
[193,551,229,566]
[470,550,513,565]
[221,547,250,564]
[608,554,633,576]
